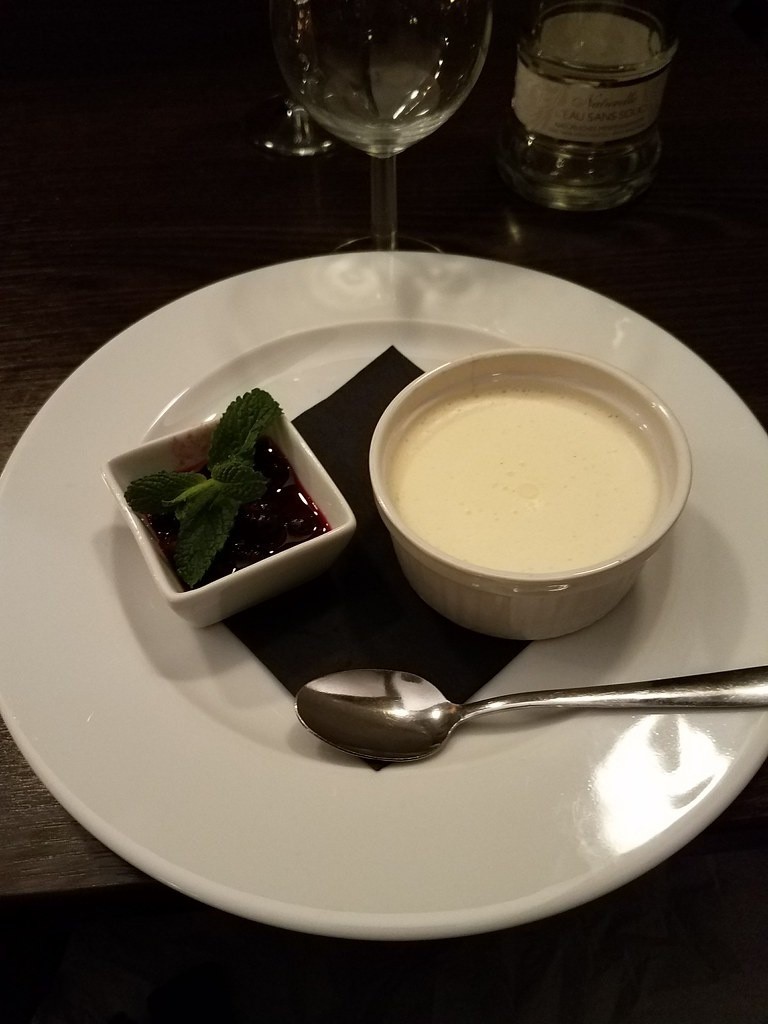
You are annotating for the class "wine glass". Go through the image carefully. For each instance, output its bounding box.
[267,0,492,252]
[246,87,339,157]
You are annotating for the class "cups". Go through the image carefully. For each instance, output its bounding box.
[509,2,679,213]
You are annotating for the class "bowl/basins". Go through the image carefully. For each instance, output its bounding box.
[369,351,693,639]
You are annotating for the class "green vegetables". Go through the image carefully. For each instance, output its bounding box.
[124,388,284,590]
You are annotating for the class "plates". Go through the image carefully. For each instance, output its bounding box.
[108,403,358,629]
[0,250,768,942]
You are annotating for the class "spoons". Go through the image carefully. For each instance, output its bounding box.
[295,667,768,760]
